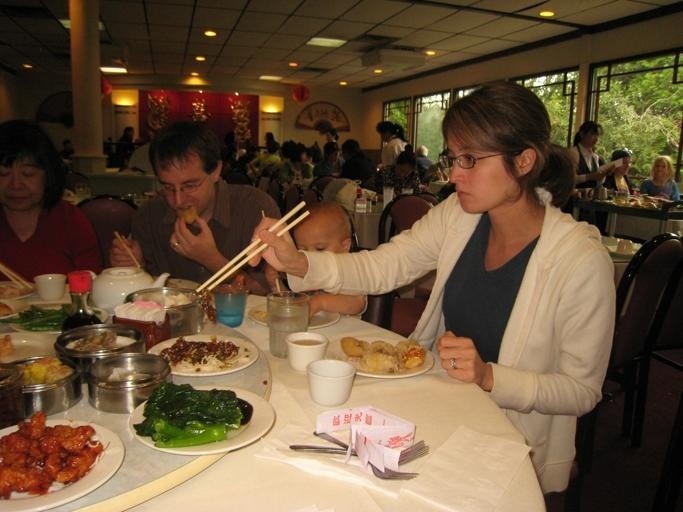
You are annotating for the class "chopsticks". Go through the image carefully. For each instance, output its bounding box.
[113,231,145,273]
[196,202,310,297]
[247,297,343,329]
[1,262,31,291]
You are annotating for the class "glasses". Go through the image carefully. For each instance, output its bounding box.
[437,147,504,170]
[156,174,208,193]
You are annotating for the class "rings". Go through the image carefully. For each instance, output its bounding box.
[451,359,456,369]
[173,242,180,248]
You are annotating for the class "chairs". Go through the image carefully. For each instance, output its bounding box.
[564,200,683,511]
[338,192,439,337]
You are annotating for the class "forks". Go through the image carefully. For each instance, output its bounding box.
[288,430,428,481]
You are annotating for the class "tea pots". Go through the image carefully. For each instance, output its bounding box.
[81,267,172,314]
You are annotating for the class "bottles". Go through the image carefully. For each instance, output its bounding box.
[65,184,149,205]
[366,197,371,213]
[353,189,367,214]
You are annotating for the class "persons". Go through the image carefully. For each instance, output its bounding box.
[246,82,617,494]
[111,119,280,295]
[536,122,681,214]
[266,199,368,317]
[121,120,452,196]
[2,120,100,282]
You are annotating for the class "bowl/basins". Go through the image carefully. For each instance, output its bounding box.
[114,288,202,349]
[8,326,172,418]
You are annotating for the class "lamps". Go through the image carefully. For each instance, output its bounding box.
[98,45,131,75]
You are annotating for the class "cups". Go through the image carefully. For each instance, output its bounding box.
[598,187,606,200]
[283,330,357,406]
[268,289,309,357]
[0,364,23,430]
[212,279,247,327]
[584,188,593,201]
[34,273,68,300]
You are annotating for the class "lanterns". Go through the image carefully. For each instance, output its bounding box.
[292,85,311,105]
[101,77,112,100]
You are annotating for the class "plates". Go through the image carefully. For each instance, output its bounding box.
[8,301,109,335]
[0,419,124,512]
[148,333,261,377]
[335,334,437,379]
[129,384,276,456]
[1,282,36,319]
[589,230,640,257]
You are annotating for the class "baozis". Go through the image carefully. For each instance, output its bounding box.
[16,357,72,385]
[163,293,191,306]
